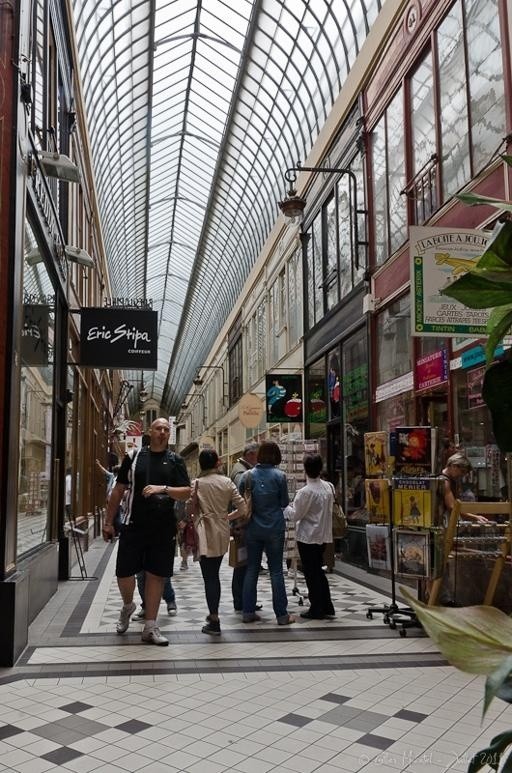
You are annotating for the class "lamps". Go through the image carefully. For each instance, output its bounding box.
[172,413,192,438]
[64,244,96,269]
[276,160,359,272]
[193,365,225,406]
[36,149,82,183]
[181,393,205,426]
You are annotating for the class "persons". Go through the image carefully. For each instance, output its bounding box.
[65,466,80,512]
[437,454,488,527]
[95,416,338,645]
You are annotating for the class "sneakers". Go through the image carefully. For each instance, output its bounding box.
[276,614,297,625]
[201,611,222,636]
[300,607,336,620]
[116,602,177,646]
[180,561,188,570]
[235,604,263,623]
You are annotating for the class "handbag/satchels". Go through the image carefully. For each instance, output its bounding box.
[183,519,198,548]
[232,470,253,528]
[229,528,250,568]
[329,483,348,539]
